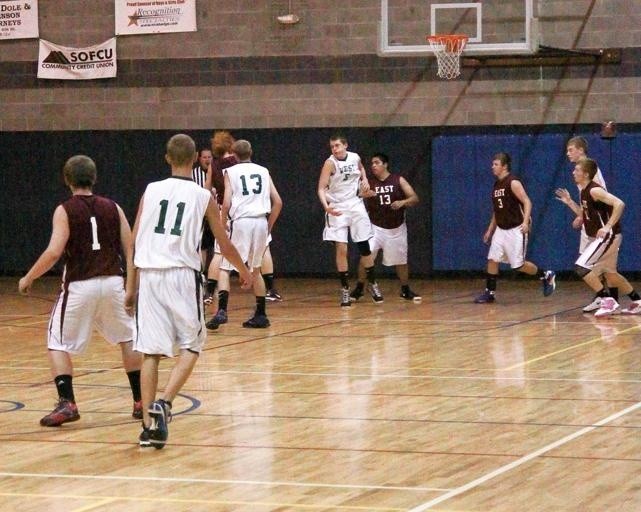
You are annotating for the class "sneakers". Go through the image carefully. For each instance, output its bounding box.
[621,299,641,314]
[594,297,620,316]
[265,289,281,301]
[205,309,227,330]
[340,286,351,307]
[399,290,422,300]
[582,296,602,312]
[133,398,172,450]
[243,314,270,328]
[350,289,364,302]
[40,400,80,426]
[475,288,496,303]
[367,281,384,304]
[203,293,214,305]
[540,270,556,297]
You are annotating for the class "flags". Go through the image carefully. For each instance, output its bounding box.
[35,35,117,82]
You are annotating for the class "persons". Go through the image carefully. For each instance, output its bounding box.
[190,129,283,330]
[348,151,423,305]
[121,133,255,451]
[565,134,624,314]
[474,150,557,306]
[315,131,384,312]
[553,157,641,320]
[15,152,143,429]
